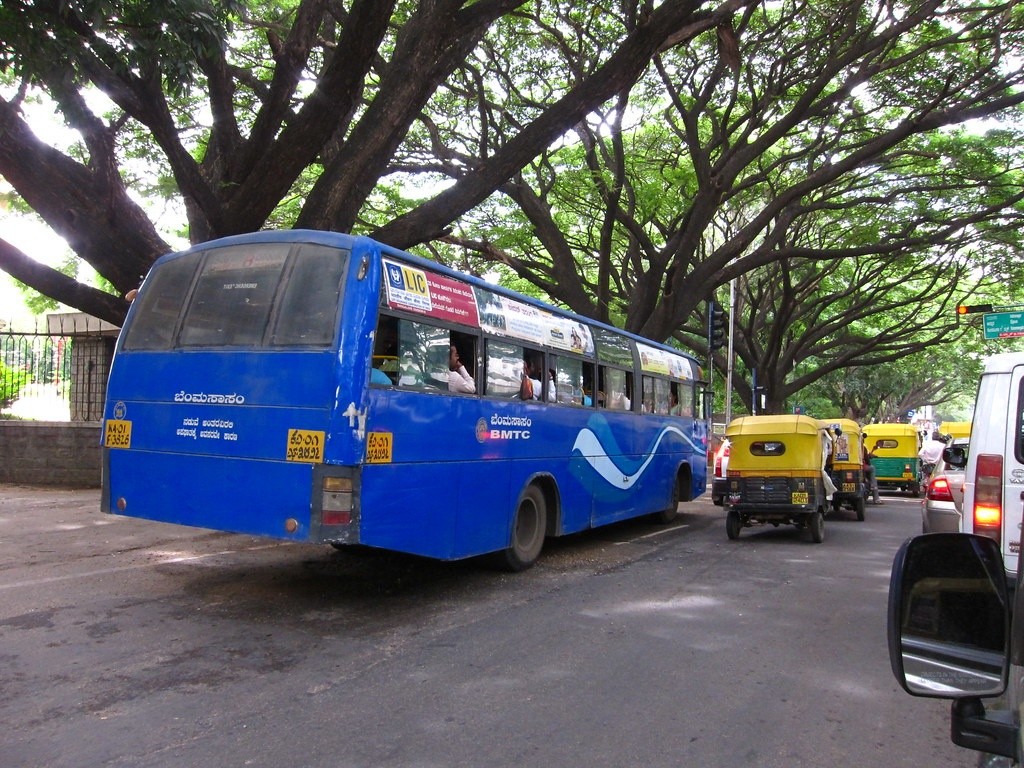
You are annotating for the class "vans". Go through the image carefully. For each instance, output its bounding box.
[941,352,1024,592]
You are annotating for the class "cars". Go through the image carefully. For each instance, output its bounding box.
[711,438,731,506]
[920,437,970,533]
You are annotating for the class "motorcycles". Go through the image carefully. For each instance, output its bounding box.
[816,418,882,521]
[862,421,928,497]
[724,414,843,542]
[922,420,972,498]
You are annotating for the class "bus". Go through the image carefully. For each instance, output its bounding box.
[98,227,717,574]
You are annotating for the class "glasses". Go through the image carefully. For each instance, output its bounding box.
[452,353,459,359]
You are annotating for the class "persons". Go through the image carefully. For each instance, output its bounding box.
[569,372,593,407]
[524,360,557,403]
[427,341,477,394]
[658,390,682,416]
[917,429,953,487]
[860,433,884,505]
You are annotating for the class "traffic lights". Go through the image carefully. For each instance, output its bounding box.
[708,310,726,351]
[957,303,993,314]
